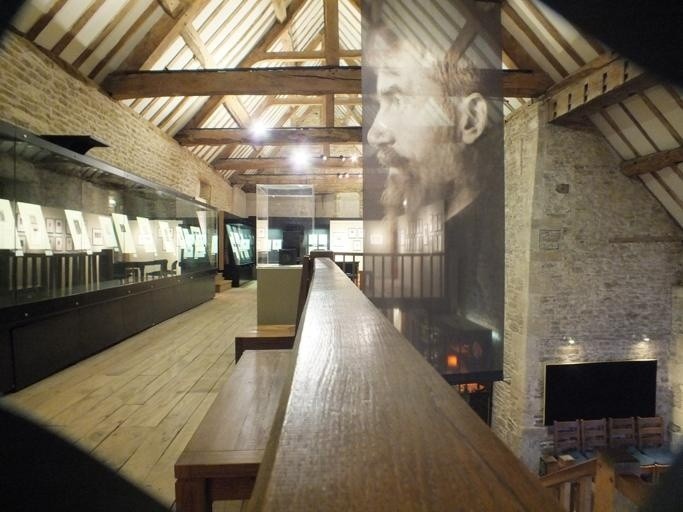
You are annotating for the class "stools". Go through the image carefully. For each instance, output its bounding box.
[230,319,299,352]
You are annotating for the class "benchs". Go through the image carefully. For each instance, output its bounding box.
[170,347,295,511]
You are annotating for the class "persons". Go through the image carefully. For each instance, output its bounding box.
[367,25,503,338]
